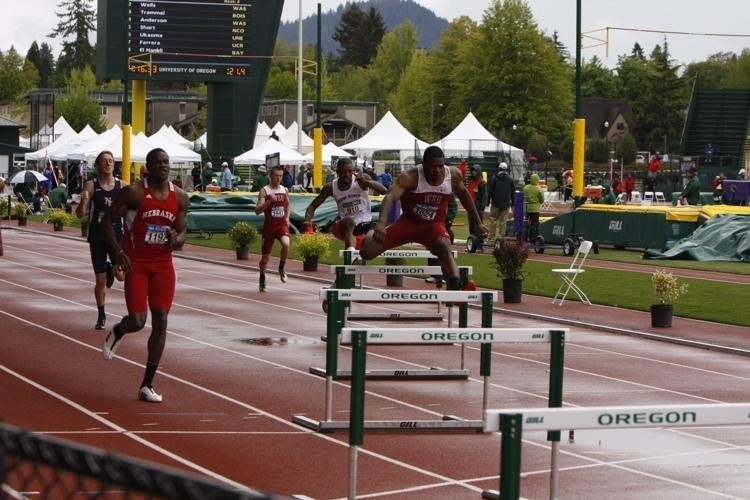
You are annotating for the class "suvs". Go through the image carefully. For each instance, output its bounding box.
[612,155,645,163]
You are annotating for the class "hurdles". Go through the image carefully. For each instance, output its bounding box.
[321,265,474,345]
[338,247,459,321]
[309,264,474,380]
[342,327,567,499]
[482,404,750,500]
[292,288,498,432]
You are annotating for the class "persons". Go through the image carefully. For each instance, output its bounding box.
[221,162,233,192]
[486,162,515,242]
[613,175,623,199]
[679,166,701,205]
[562,174,572,202]
[711,173,727,202]
[467,164,486,238]
[172,174,182,190]
[47,183,68,209]
[624,173,635,202]
[202,162,218,192]
[256,166,290,294]
[297,165,305,185]
[301,157,391,315]
[522,174,545,244]
[704,143,715,165]
[13,183,33,202]
[282,164,293,188]
[378,168,392,190]
[648,153,661,179]
[76,150,131,331]
[349,146,487,307]
[101,148,190,402]
[250,165,270,192]
[303,163,313,190]
[191,161,202,191]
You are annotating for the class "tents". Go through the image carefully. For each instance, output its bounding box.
[148,122,193,149]
[431,111,524,188]
[262,119,286,144]
[23,129,73,171]
[193,131,207,149]
[48,123,98,190]
[280,121,324,156]
[253,120,271,148]
[232,137,304,188]
[131,132,203,192]
[66,123,133,196]
[41,113,75,146]
[303,141,368,171]
[128,130,147,162]
[338,109,430,168]
[18,122,49,148]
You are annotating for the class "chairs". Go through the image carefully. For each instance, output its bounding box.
[43,195,61,224]
[551,241,593,307]
[616,193,623,204]
[543,192,557,210]
[541,192,550,209]
[632,191,640,198]
[17,192,33,216]
[644,192,653,205]
[655,192,665,205]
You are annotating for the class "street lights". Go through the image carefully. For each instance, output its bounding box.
[429,103,444,140]
[605,121,609,164]
[511,123,517,148]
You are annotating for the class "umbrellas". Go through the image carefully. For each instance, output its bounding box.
[4,169,49,184]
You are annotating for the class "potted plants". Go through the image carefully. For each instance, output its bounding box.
[650,268,689,327]
[488,239,531,304]
[48,208,72,231]
[228,222,258,260]
[15,204,27,226]
[293,225,336,272]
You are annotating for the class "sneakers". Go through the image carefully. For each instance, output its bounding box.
[106,264,114,288]
[280,270,288,282]
[104,329,121,360]
[140,385,163,402]
[259,279,267,291]
[454,281,476,306]
[345,247,358,265]
[95,315,107,330]
[322,283,335,313]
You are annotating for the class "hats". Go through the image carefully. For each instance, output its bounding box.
[220,162,229,167]
[206,162,212,168]
[499,162,508,169]
[258,166,266,172]
[685,166,698,175]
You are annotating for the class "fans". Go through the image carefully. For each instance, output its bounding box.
[386,242,405,285]
